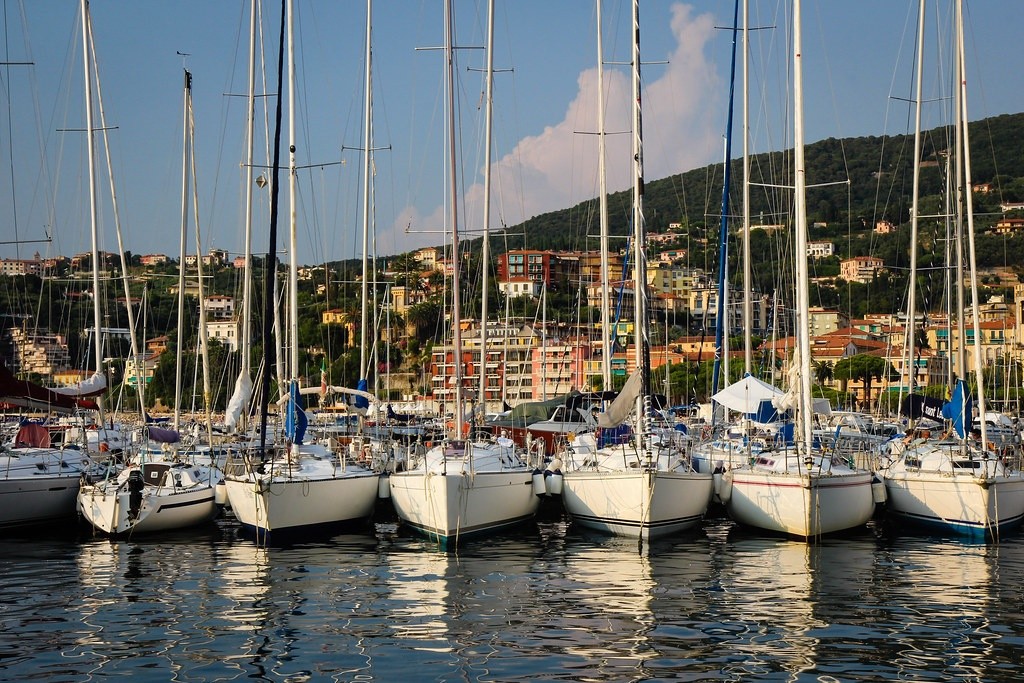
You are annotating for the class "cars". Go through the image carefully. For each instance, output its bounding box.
[362,410,509,430]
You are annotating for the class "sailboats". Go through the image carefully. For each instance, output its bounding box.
[544,5,715,539]
[5,1,1024,545]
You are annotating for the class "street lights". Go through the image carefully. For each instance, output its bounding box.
[403,228,515,431]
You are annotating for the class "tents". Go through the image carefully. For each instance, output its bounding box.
[710,375,785,413]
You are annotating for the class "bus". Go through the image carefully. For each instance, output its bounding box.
[303,407,350,424]
